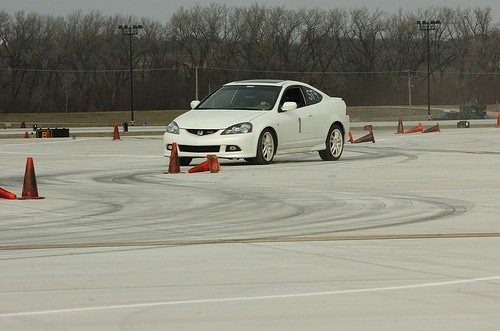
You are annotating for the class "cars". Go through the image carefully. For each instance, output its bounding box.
[162,78,351,164]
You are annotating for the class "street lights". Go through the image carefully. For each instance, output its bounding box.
[417,20,441,121]
[118,24,144,126]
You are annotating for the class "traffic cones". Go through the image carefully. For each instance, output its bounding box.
[112,120,122,140]
[404,123,423,133]
[45,130,50,138]
[353,131,375,143]
[424,123,440,133]
[0,186,16,200]
[364,125,373,132]
[23,131,29,138]
[162,142,186,173]
[18,156,45,201]
[495,112,500,128]
[394,116,405,134]
[188,154,221,173]
[348,131,353,143]
[20,121,26,128]
